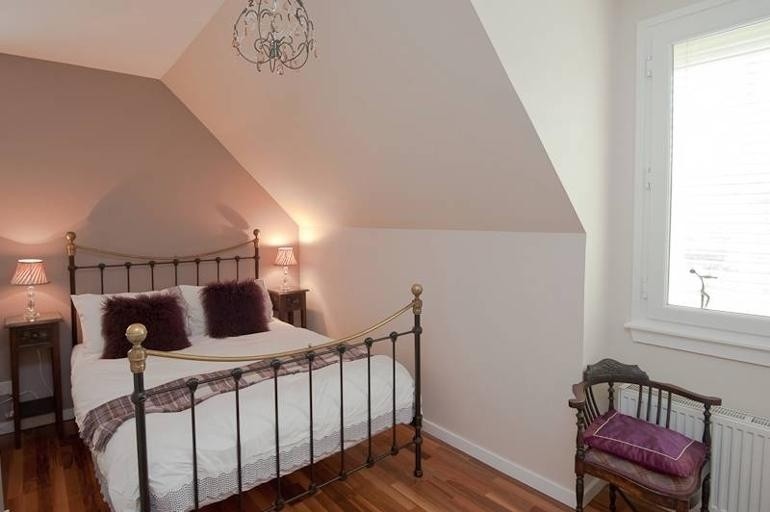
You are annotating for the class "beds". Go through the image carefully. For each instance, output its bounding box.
[66,227,423,512]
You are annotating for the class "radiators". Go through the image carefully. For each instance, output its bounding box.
[618,384,770,512]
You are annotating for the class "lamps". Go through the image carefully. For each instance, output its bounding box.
[273,246,297,292]
[232,0,318,76]
[8,259,51,319]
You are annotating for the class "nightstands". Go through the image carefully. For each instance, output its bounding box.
[3,311,66,449]
[267,286,310,329]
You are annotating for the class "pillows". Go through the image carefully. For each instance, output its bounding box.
[70,279,274,360]
[581,408,706,478]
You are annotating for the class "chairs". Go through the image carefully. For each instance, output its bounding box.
[569,358,722,512]
